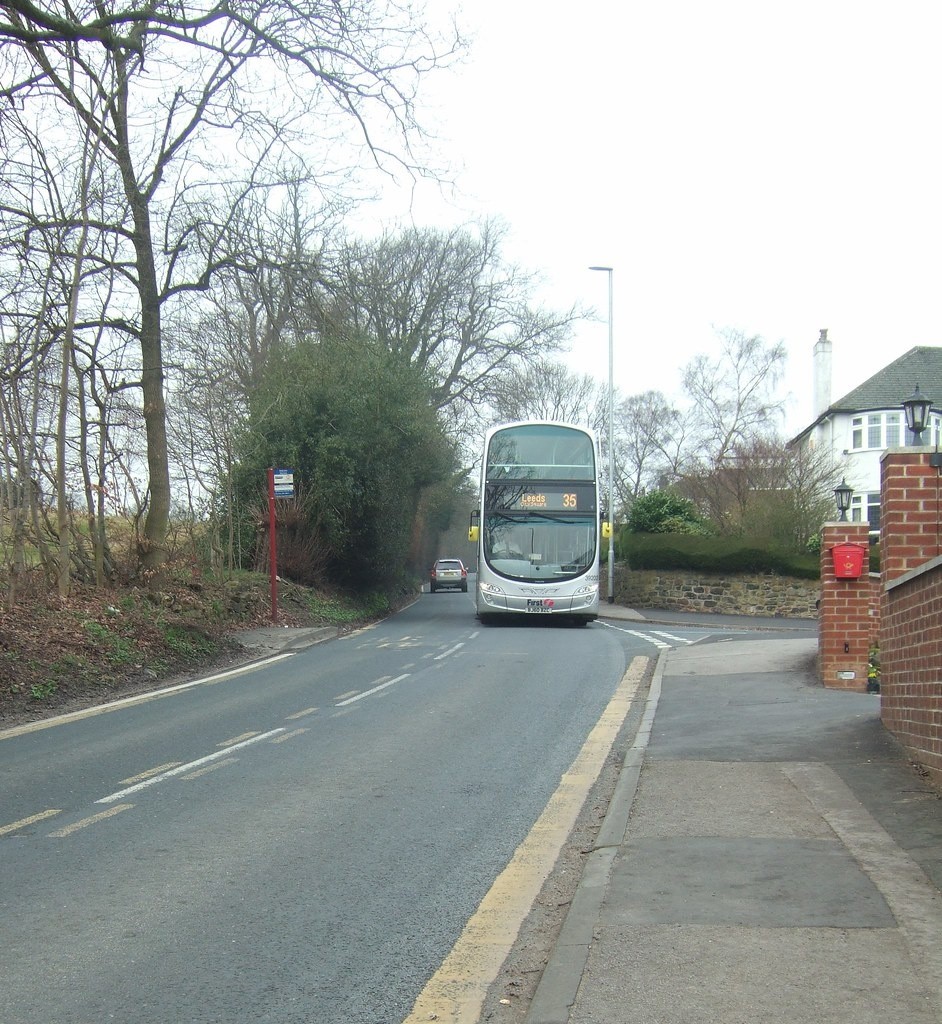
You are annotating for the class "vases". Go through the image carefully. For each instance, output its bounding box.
[868,678,879,691]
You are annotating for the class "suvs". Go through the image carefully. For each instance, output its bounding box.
[428,558,469,593]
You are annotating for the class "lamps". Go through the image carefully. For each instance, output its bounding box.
[832,477,859,522]
[900,383,934,446]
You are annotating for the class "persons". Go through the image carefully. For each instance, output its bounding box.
[492,531,523,555]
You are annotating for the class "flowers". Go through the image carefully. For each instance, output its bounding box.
[867,664,879,678]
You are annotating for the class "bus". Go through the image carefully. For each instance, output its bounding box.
[465,419,601,624]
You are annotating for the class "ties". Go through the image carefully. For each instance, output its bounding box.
[506,542,509,553]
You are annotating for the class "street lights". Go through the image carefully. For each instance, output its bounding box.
[588,263,616,604]
